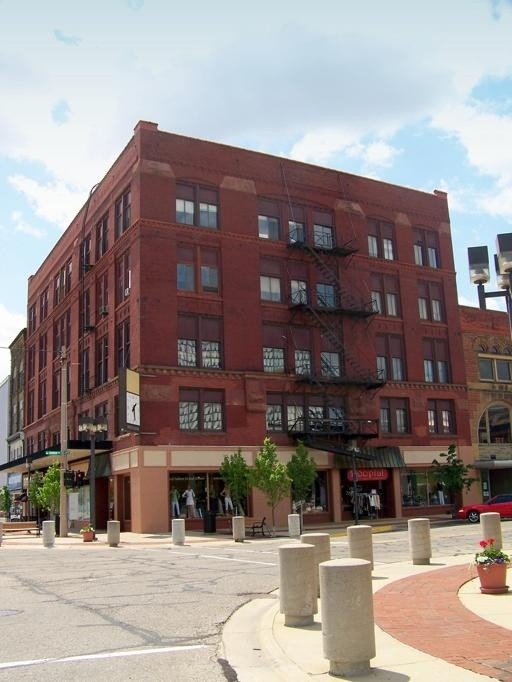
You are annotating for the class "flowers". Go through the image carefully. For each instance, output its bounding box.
[471,537,510,571]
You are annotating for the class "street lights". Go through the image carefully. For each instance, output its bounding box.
[78,416,109,530]
[467,233,512,332]
[26,457,32,495]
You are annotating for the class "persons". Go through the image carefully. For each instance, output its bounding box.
[169,484,181,517]
[182,485,197,519]
[220,489,234,516]
[437,482,444,504]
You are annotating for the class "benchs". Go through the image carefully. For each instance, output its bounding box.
[2,521,41,535]
[243,517,265,535]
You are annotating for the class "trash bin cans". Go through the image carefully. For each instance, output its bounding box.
[202,510,216,533]
[55,513,60,535]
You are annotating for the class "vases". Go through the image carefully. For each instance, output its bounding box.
[476,564,508,594]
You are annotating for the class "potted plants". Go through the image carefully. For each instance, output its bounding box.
[79,526,96,541]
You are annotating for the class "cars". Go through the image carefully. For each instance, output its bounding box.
[456,493,512,523]
